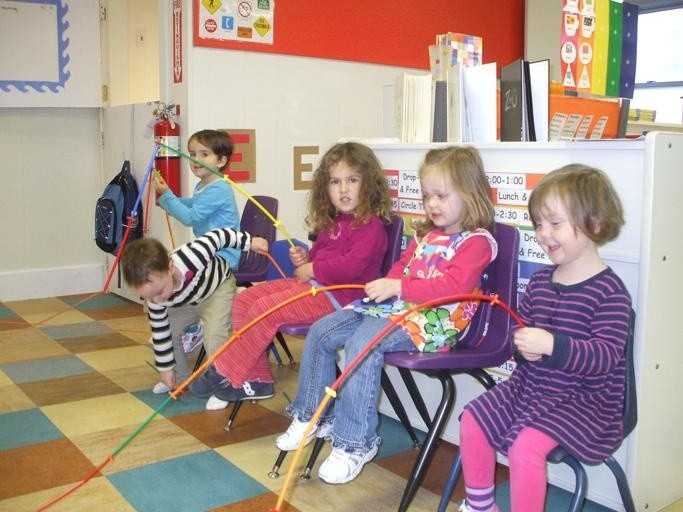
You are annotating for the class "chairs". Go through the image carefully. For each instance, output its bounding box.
[437,306,637,512]
[191,194,283,374]
[302,222,526,512]
[222,214,421,478]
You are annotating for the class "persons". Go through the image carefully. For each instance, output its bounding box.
[121,227,269,410]
[459,161,631,512]
[189,144,392,402]
[276,147,497,484]
[147,129,240,354]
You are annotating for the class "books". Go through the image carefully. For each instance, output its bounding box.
[396,33,550,142]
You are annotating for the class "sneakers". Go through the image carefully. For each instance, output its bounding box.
[319,443,379,485]
[456,498,499,512]
[180,321,204,353]
[189,363,232,396]
[276,415,337,451]
[215,381,274,400]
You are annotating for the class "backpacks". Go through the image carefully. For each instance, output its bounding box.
[94,161,143,256]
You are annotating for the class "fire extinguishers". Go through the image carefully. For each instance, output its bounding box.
[155,105,181,207]
